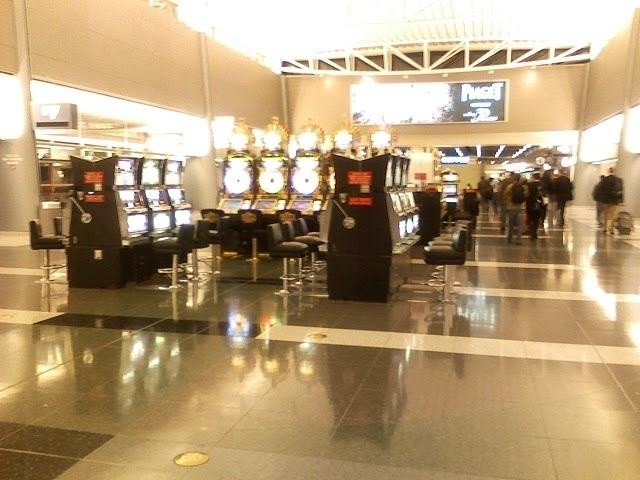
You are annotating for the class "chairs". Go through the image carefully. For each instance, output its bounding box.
[152,208,325,293]
[423,220,472,305]
[29,216,69,282]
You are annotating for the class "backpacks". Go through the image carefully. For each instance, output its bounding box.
[512,184,525,204]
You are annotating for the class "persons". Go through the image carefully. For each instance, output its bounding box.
[476,167,625,246]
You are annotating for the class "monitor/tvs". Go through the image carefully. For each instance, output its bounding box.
[388,190,419,237]
[442,184,457,196]
[117,186,192,234]
[221,198,313,210]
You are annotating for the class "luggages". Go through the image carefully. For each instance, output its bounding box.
[617,203,633,234]
